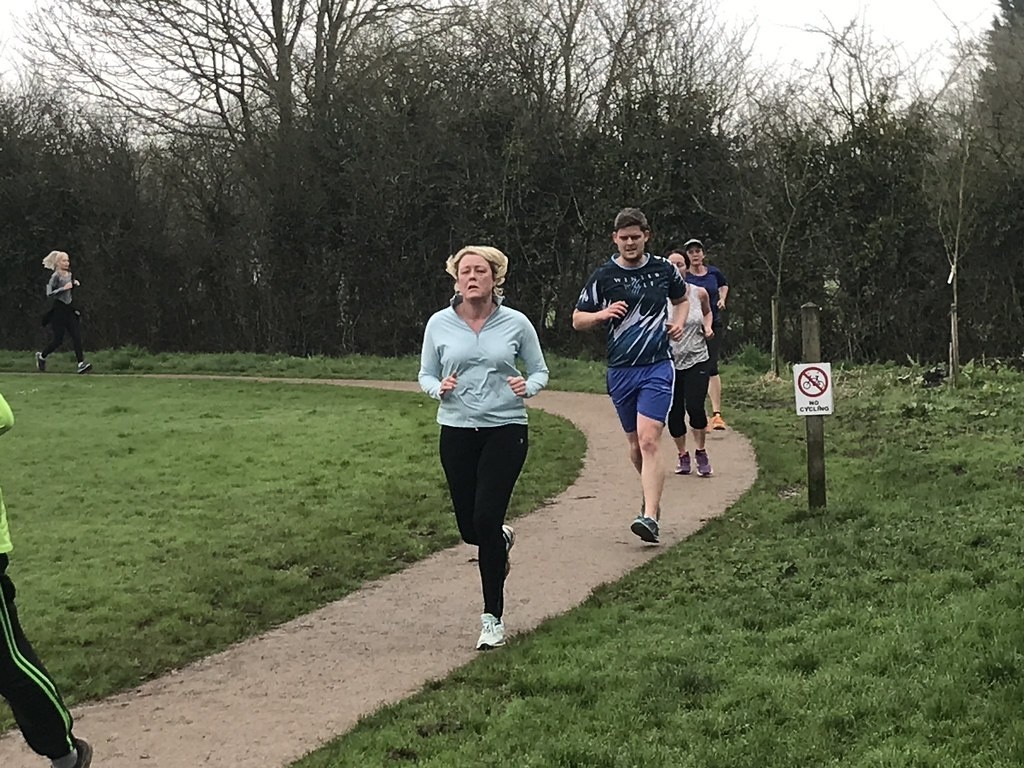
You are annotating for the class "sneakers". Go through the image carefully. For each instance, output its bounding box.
[641,489,661,522]
[77,362,92,375]
[35,352,47,371]
[476,613,506,650]
[704,416,711,434]
[694,448,713,477]
[502,524,515,580]
[630,515,659,543]
[674,452,692,474]
[712,413,726,430]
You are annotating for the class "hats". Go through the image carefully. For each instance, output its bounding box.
[684,240,704,252]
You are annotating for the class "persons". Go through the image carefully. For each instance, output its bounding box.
[0,393,93,768]
[572,208,690,543]
[418,245,550,651]
[36,250,92,373]
[664,249,715,476]
[684,238,729,433]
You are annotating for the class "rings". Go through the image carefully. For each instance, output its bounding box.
[518,388,520,392]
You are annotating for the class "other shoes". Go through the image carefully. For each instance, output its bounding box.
[74,738,93,768]
